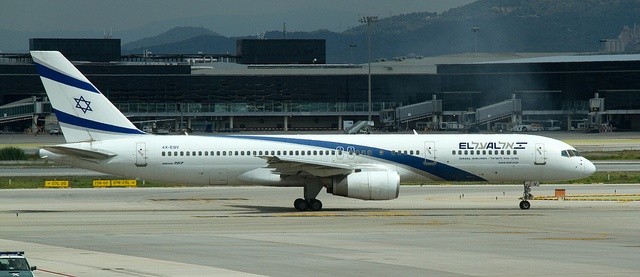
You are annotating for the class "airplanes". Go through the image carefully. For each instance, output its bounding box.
[29,51,596,210]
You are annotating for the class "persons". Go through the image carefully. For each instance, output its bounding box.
[15,258,30,269]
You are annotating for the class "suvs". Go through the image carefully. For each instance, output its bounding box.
[1,250,36,276]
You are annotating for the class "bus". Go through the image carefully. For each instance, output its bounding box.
[416,115,616,133]
[140,118,175,133]
